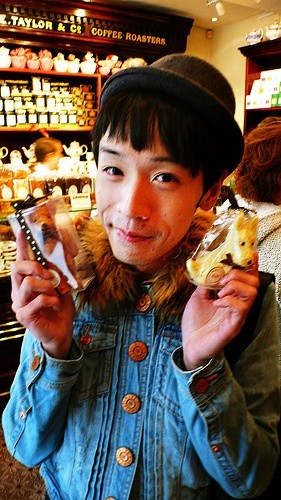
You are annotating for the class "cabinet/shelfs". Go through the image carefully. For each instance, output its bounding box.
[0,0,195,396]
[244,46,281,134]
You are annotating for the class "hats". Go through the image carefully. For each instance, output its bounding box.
[100,53,245,177]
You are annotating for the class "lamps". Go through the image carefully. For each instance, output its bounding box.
[207,0,225,16]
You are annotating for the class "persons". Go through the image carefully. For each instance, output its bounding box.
[214,118,281,312]
[1,55,281,500]
[30,137,64,178]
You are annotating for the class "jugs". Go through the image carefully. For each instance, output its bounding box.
[22,142,38,162]
[62,141,88,161]
[245,28,263,45]
[265,21,281,40]
[0,146,8,166]
